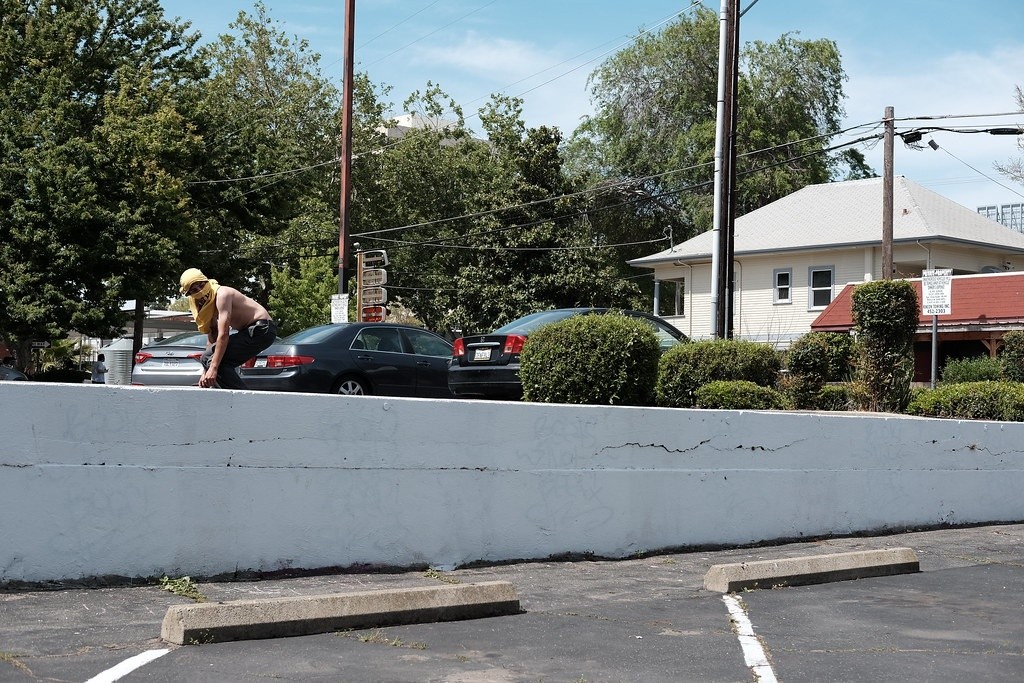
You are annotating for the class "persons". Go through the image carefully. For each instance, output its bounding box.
[91,354,108,385]
[178,268,277,392]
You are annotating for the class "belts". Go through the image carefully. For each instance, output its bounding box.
[248,319,275,326]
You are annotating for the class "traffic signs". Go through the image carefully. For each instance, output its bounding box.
[30,339,51,348]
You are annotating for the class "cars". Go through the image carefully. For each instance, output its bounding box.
[232,322,454,398]
[447,307,697,403]
[131,328,284,389]
[0,363,29,381]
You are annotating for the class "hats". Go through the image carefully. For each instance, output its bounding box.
[179,268,209,295]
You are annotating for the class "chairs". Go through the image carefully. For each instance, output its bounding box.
[351,337,364,350]
[378,334,401,353]
